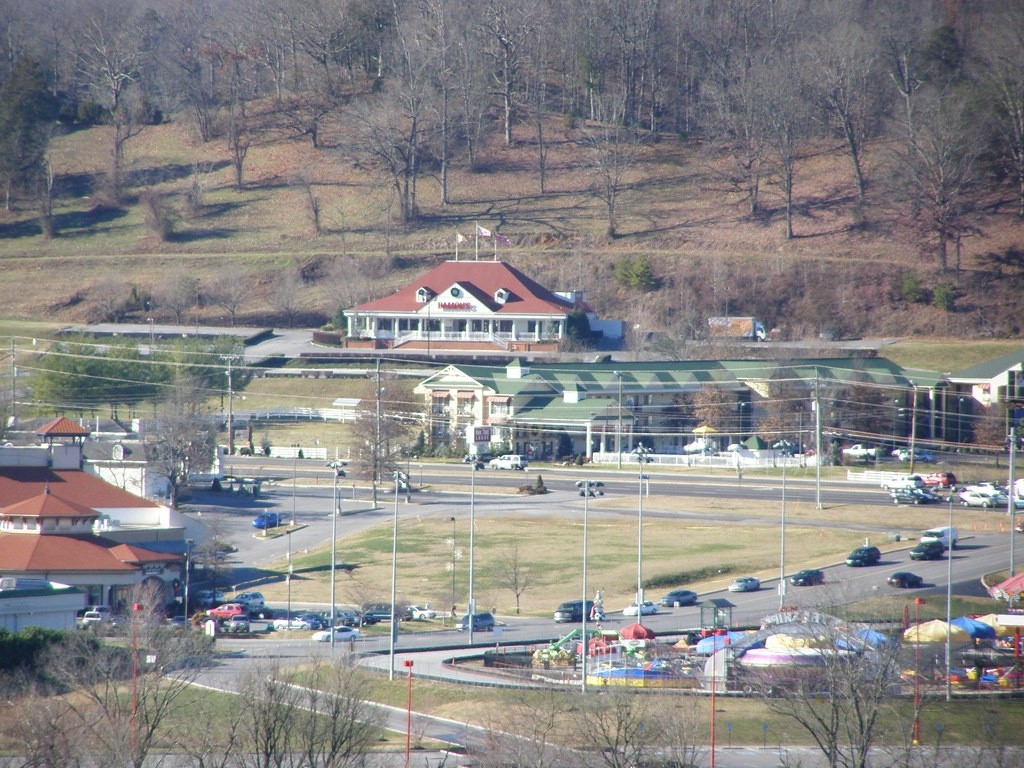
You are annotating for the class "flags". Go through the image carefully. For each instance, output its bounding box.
[458,225,509,245]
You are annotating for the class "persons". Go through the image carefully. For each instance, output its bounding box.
[427,601,433,609]
[192,608,203,633]
[336,469,339,483]
[452,603,456,616]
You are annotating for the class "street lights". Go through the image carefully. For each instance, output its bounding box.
[945,486,969,704]
[912,597,927,743]
[183,539,197,630]
[131,602,144,747]
[401,659,415,768]
[325,459,349,648]
[390,469,411,681]
[574,480,602,699]
[701,628,731,768]
[628,442,655,640]
[1008,426,1016,579]
[772,438,796,613]
[909,379,920,476]
[468,458,485,646]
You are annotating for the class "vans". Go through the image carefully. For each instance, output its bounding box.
[490,453,528,470]
[556,600,598,624]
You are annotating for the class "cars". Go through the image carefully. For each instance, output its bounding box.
[454,612,497,635]
[83,511,437,647]
[728,576,759,594]
[771,435,1024,601]
[324,458,350,468]
[622,588,698,619]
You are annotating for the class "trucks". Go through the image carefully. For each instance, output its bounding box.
[700,314,846,343]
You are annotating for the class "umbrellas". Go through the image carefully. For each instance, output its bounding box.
[576,623,655,657]
[837,573,1024,667]
